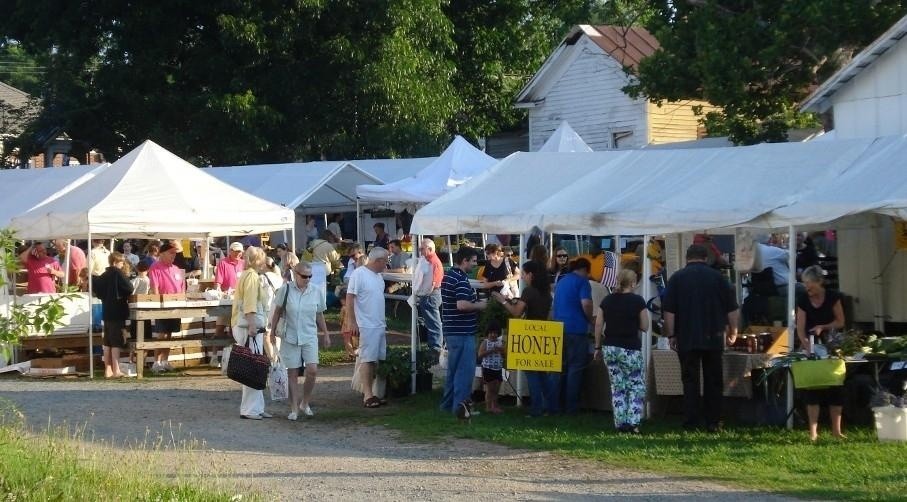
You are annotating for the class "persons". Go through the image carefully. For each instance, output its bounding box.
[0,212,851,443]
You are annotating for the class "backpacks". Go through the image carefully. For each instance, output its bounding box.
[301,245,314,263]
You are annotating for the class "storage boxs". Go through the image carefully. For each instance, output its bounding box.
[127,292,225,369]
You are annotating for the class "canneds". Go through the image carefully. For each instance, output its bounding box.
[758,332,772,354]
[746,335,758,353]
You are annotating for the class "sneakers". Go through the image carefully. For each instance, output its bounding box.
[210,359,222,368]
[162,361,174,372]
[151,363,165,372]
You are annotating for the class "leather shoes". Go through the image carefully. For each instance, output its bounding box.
[240,414,263,420]
[287,412,299,421]
[260,413,273,419]
[299,402,313,417]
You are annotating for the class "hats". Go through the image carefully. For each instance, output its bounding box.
[230,242,244,252]
[160,244,175,253]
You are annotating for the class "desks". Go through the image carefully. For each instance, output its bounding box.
[382,272,485,294]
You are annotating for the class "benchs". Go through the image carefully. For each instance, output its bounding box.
[384,294,411,319]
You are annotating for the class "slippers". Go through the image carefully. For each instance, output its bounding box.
[375,396,388,405]
[365,398,382,409]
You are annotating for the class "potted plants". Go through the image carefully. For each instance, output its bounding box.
[409,347,439,393]
[376,345,411,398]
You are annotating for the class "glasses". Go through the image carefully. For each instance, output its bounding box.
[471,260,478,264]
[294,270,313,280]
[505,254,514,257]
[555,254,569,258]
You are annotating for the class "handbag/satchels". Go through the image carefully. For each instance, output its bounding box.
[226,334,271,391]
[506,256,522,300]
[266,344,290,401]
[185,272,198,292]
[273,284,290,338]
